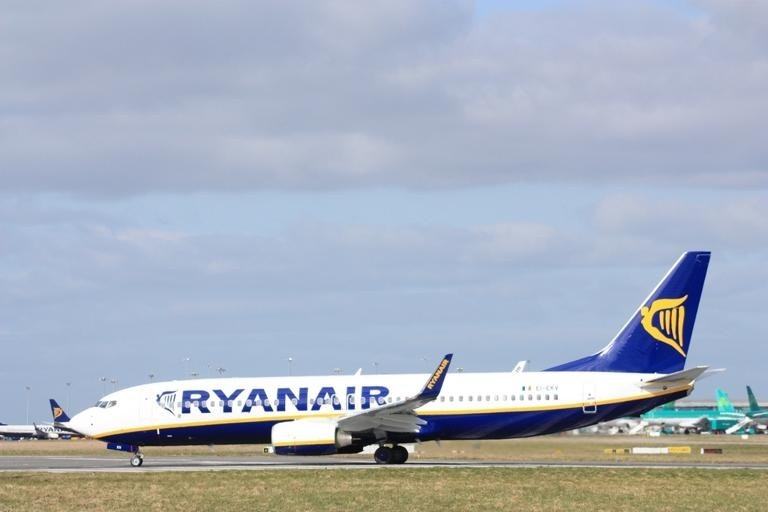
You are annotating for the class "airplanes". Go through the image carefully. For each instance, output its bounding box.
[578,387,767,436]
[66,250,728,462]
[0,398,84,441]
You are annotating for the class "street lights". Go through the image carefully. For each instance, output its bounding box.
[24,383,33,424]
[67,383,72,417]
[98,375,107,397]
[187,355,474,380]
[110,378,116,393]
[148,371,154,383]
